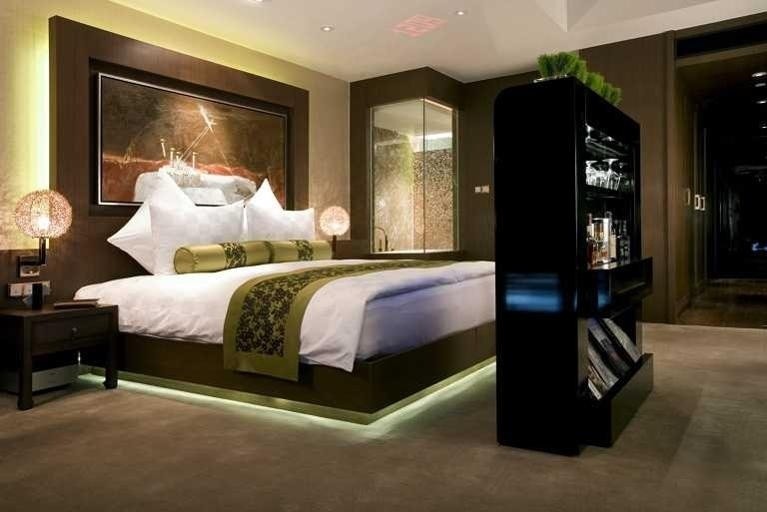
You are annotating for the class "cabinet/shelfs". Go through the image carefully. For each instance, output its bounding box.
[490,76,662,455]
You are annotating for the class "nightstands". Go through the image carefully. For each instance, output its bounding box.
[0,299,121,412]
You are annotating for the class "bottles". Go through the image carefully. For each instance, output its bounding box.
[585,211,629,268]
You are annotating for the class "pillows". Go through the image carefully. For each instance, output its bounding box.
[243,177,312,242]
[141,190,244,278]
[263,238,333,264]
[172,239,273,277]
[102,170,196,276]
[282,206,317,240]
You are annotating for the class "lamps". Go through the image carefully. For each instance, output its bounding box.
[317,205,353,254]
[12,188,74,278]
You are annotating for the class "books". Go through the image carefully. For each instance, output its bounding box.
[53,298,98,309]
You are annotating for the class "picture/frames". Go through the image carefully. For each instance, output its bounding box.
[86,55,295,217]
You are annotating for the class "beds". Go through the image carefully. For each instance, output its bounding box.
[68,257,495,427]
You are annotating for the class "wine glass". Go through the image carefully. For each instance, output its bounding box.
[583,156,628,189]
